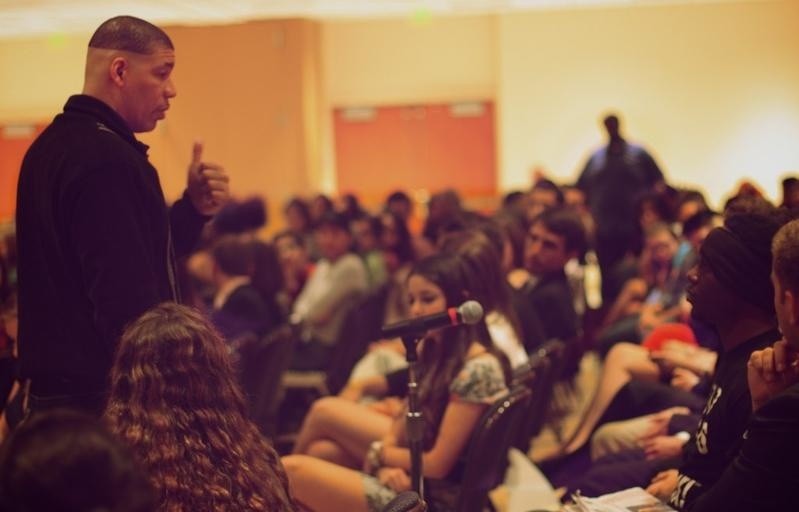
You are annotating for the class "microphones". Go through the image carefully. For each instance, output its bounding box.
[381,299,484,339]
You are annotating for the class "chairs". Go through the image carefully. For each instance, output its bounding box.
[426,334,563,506]
[235,274,386,445]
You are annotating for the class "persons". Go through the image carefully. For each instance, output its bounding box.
[0,178,799,512]
[575,118,667,249]
[16,16,231,418]
